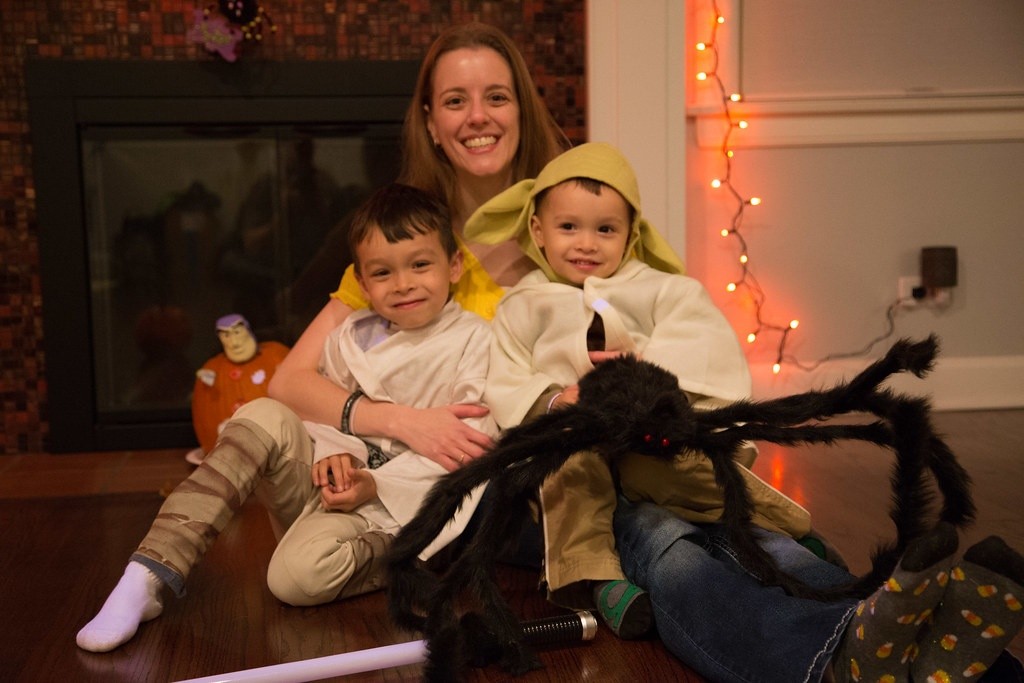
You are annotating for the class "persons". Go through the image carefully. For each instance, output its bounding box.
[481,142,851,639]
[77,183,511,652]
[267,23,1023,683]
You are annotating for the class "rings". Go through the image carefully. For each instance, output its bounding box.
[458,453,465,463]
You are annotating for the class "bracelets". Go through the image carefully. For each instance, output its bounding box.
[342,390,363,434]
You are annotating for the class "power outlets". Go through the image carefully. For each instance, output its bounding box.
[895,276,955,307]
